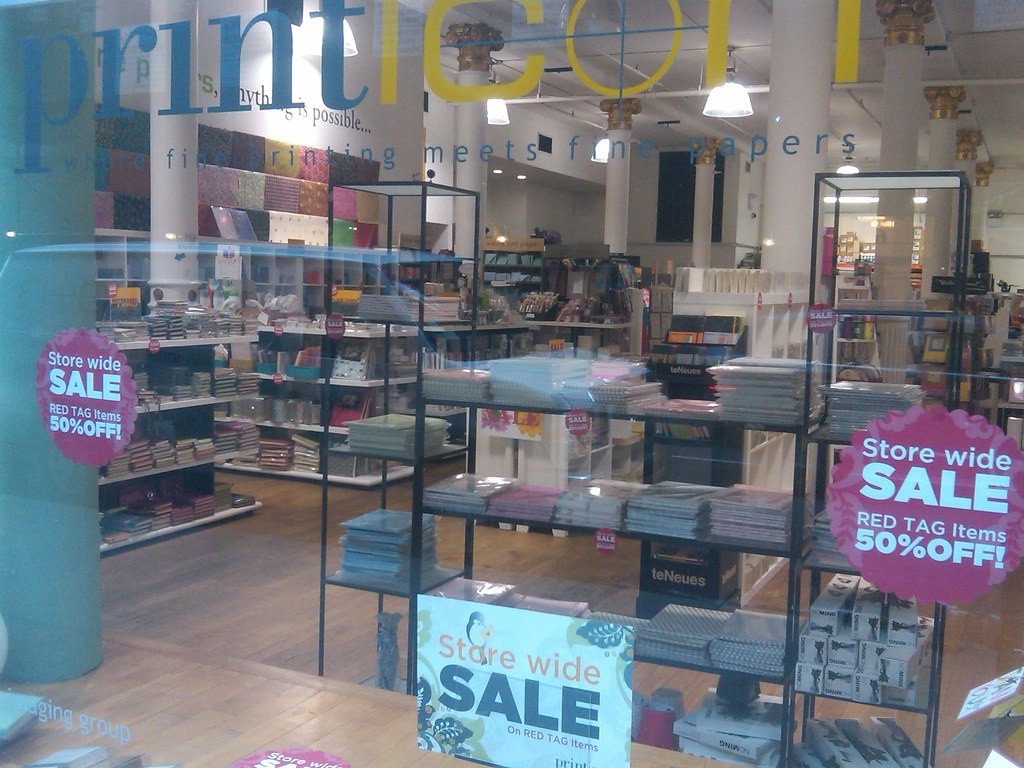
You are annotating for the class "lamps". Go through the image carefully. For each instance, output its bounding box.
[702,45,754,118]
[486,56,511,126]
[292,10,358,57]
[591,139,610,164]
[837,144,859,174]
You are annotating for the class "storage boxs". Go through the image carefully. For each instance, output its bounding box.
[672,573,935,767]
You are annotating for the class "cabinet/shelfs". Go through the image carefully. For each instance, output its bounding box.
[406,378,828,768]
[317,181,480,697]
[632,221,1023,621]
[94,226,809,622]
[781,171,970,768]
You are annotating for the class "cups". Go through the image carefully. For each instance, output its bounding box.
[838,316,876,339]
